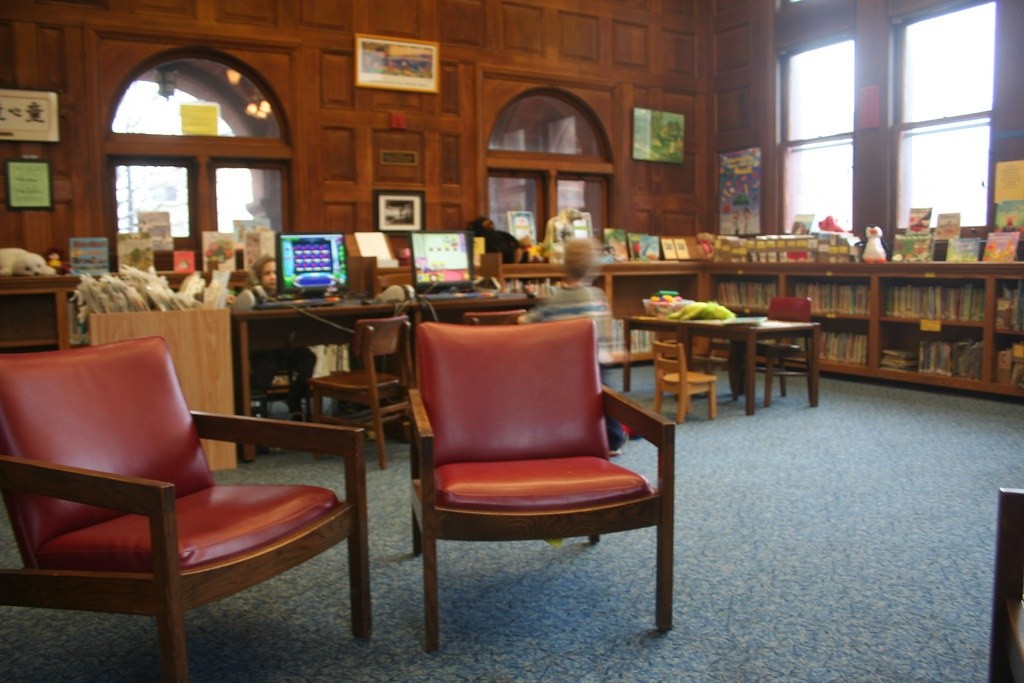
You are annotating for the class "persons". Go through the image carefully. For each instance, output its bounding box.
[521,241,629,457]
[243,256,318,421]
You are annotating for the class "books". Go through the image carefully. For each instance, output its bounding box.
[64,210,279,275]
[710,273,1023,384]
[614,320,659,356]
[595,208,1023,266]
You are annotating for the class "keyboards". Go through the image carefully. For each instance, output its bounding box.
[252,299,335,310]
[419,292,501,301]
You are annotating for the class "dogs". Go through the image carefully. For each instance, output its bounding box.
[0,248,56,277]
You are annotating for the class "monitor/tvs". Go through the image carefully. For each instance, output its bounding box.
[409,230,472,293]
[276,232,350,299]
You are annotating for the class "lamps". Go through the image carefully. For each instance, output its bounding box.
[226,69,243,85]
[152,66,177,100]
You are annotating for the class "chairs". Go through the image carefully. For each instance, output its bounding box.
[0,296,813,683]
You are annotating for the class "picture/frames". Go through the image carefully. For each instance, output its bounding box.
[0,85,61,146]
[714,145,765,237]
[3,159,55,211]
[354,33,440,94]
[632,107,684,164]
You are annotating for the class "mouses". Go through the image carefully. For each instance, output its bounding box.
[527,291,538,298]
[362,299,377,305]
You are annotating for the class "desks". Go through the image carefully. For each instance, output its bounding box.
[622,316,822,415]
[230,299,539,463]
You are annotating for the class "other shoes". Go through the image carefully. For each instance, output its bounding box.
[609,430,628,455]
[286,399,304,421]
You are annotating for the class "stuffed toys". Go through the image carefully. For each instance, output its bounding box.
[855,223,891,263]
[0,247,55,279]
[669,300,738,321]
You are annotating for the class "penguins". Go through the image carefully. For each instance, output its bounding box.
[854,224,892,263]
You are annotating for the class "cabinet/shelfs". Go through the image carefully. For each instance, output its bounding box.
[0,272,246,351]
[472,252,1024,398]
